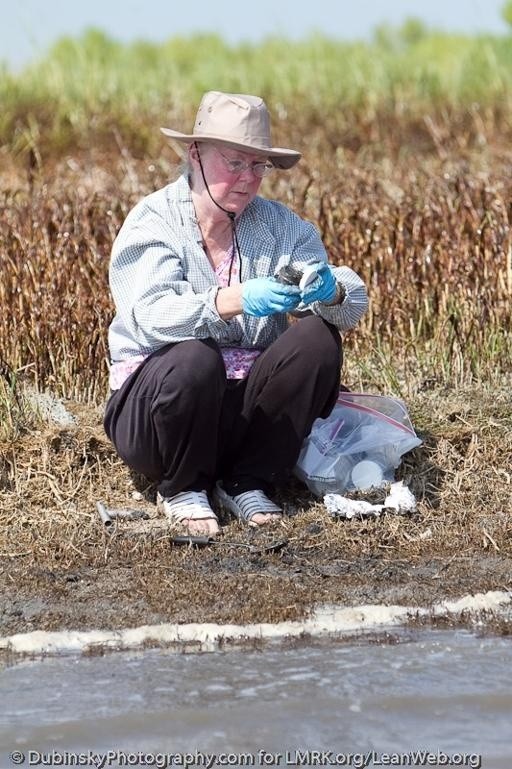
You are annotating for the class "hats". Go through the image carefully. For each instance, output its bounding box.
[157,89,302,170]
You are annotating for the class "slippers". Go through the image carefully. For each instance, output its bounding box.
[155,489,219,536]
[212,480,283,531]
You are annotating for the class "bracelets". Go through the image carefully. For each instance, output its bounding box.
[331,281,347,307]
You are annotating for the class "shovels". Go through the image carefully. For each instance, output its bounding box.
[170,535,290,552]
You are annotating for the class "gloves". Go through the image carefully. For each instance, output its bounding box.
[300,261,337,306]
[242,277,301,317]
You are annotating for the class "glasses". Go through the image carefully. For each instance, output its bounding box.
[210,142,275,178]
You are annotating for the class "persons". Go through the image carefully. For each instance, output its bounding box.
[105,90,371,547]
